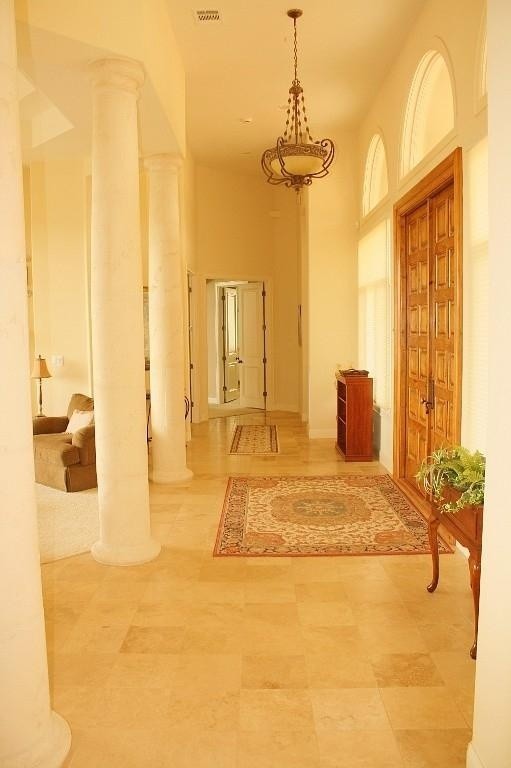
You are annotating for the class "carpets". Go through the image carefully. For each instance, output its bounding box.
[213,474,457,558]
[33,478,102,564]
[227,423,280,456]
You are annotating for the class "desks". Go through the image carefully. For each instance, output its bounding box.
[427,467,485,660]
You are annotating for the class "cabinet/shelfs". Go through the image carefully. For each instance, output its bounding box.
[336,373,375,462]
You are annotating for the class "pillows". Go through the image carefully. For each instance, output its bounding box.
[63,408,96,435]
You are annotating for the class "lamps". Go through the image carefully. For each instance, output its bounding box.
[260,6,335,197]
[32,353,53,418]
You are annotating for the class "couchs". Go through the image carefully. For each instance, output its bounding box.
[34,393,97,492]
